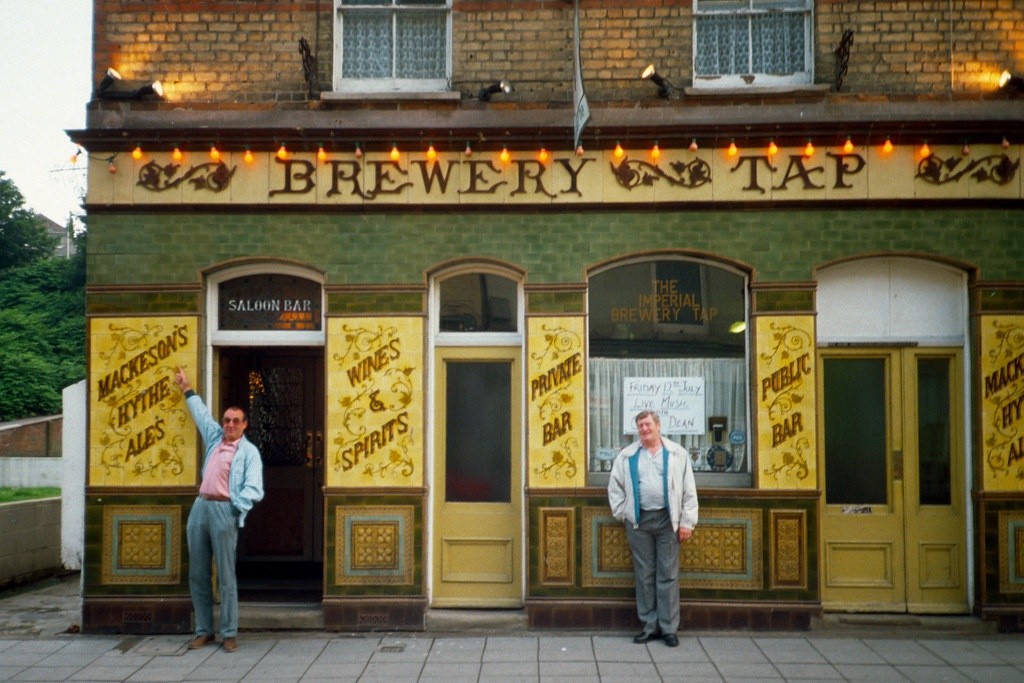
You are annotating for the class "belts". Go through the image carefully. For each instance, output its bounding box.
[198,494,231,501]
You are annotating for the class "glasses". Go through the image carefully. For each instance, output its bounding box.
[221,416,246,425]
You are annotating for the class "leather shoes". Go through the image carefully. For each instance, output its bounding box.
[634,631,662,643]
[664,634,678,647]
[188,633,215,649]
[223,637,237,652]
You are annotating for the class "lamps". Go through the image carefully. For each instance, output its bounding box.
[641,64,671,97]
[999,70,1024,91]
[98,68,120,94]
[478,80,511,101]
[135,80,163,100]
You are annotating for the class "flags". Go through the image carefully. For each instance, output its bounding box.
[573,0,593,155]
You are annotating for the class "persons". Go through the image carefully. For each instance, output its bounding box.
[607,409,700,646]
[173,362,264,652]
